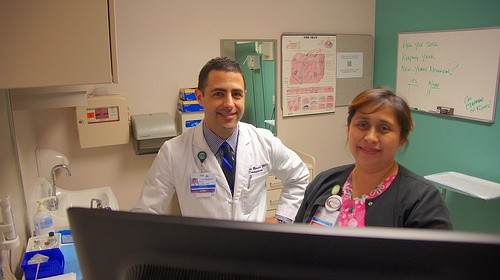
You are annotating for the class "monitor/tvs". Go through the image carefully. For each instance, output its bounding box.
[66,206,500,280]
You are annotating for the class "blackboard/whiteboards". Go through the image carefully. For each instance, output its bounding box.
[281,32,374,109]
[395,26,500,124]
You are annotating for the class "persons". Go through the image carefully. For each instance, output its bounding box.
[130,56,310,224]
[293,86,452,230]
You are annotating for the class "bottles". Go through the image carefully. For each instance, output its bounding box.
[44,241,52,249]
[48,232,58,248]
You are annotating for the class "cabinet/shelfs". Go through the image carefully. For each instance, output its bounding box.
[266,146,315,219]
[0,0,118,90]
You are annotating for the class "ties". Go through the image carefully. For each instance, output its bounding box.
[220,141,236,196]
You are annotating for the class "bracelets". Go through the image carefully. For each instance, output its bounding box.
[278,220,284,224]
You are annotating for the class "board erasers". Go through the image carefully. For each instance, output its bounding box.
[439,107,453,114]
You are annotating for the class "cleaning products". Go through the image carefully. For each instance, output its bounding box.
[32,198,53,237]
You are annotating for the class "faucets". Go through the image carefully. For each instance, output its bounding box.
[47,163,73,210]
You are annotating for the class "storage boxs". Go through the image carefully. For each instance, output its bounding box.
[21,233,65,280]
[177,88,204,113]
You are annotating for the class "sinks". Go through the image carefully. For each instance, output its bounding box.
[44,186,119,222]
